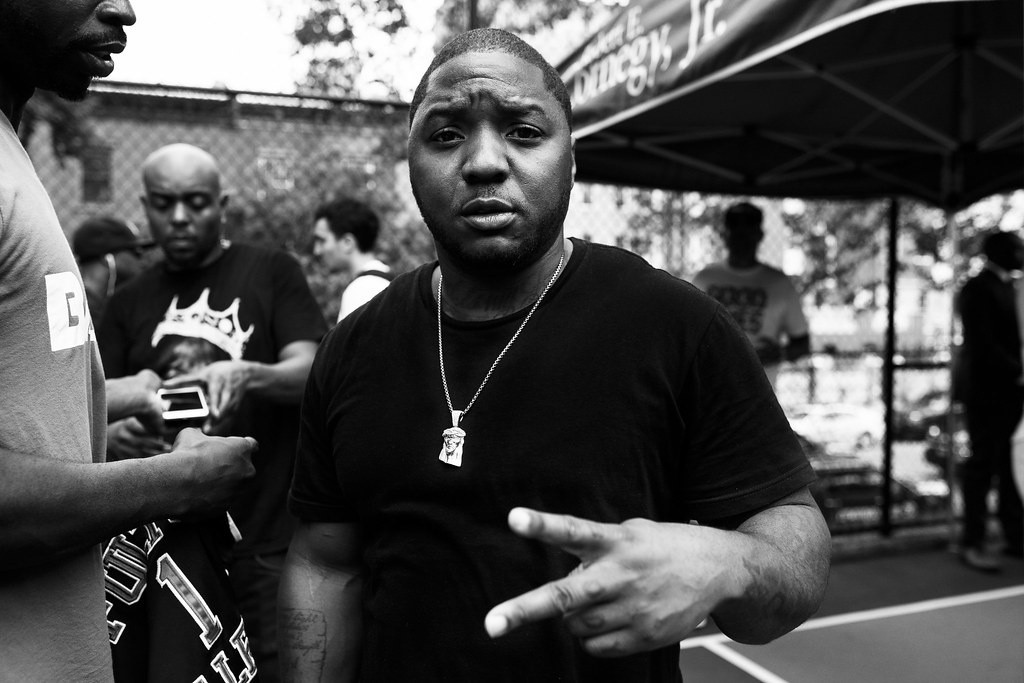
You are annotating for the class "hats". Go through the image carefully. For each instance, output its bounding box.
[72,217,157,259]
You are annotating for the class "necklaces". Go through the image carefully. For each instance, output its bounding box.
[437,253,565,468]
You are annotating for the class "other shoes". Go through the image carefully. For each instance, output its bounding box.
[949,544,1001,567]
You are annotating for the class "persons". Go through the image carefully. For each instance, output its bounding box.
[70,143,328,683]
[949,231,1024,577]
[312,200,397,325]
[280,27,833,683]
[0,0,259,683]
[691,203,810,394]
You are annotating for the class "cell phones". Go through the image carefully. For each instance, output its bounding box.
[158,385,210,424]
